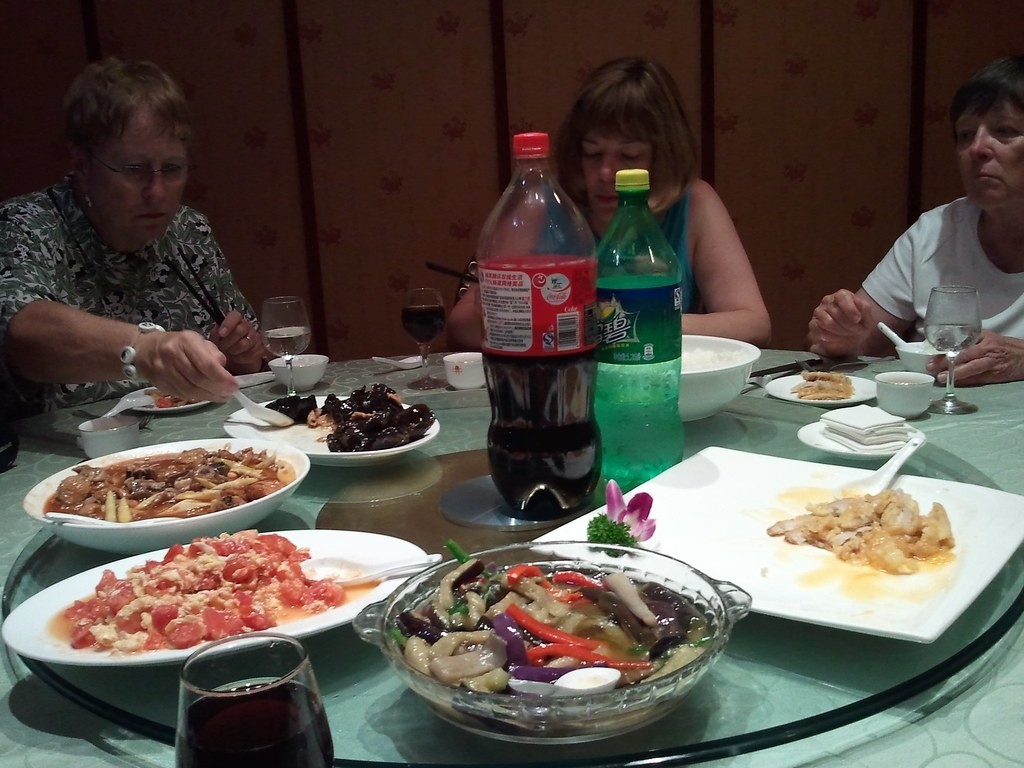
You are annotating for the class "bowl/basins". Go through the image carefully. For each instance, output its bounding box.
[351,542,751,744]
[877,343,945,420]
[442,352,487,389]
[679,334,762,422]
[269,356,329,393]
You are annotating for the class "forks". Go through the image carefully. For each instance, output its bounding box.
[794,360,869,372]
[72,409,152,431]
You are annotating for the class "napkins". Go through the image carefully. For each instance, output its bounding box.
[821,400,912,453]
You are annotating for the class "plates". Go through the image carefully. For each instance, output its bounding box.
[223,396,440,466]
[23,440,312,556]
[124,385,212,413]
[526,446,1024,643]
[767,376,920,458]
[2,530,430,666]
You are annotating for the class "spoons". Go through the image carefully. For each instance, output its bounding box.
[507,665,621,699]
[843,429,925,498]
[233,390,294,427]
[372,356,423,369]
[302,551,442,585]
[104,396,157,416]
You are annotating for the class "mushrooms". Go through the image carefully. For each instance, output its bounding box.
[396,556,705,693]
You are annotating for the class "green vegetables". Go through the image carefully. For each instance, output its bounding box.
[587,512,636,555]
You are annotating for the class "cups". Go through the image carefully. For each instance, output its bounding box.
[174,634,334,767]
[77,415,140,459]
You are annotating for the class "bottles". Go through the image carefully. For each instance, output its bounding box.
[588,169,684,507]
[475,132,595,519]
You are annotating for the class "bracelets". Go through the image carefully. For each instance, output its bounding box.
[119,322,166,380]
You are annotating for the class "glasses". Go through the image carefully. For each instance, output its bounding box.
[88,153,194,184]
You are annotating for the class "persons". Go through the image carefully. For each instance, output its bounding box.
[0,56,265,415]
[804,52,1024,388]
[446,57,772,348]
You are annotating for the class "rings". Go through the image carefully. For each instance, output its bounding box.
[245,336,253,348]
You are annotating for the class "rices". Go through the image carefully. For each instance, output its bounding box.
[680,346,752,370]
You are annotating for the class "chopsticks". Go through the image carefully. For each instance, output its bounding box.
[751,357,823,377]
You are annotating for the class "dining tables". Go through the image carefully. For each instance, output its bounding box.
[1,343,1024,764]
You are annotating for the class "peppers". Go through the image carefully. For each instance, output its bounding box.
[527,642,650,669]
[505,564,583,602]
[553,571,608,590]
[504,605,609,649]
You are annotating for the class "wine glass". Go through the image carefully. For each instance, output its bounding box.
[262,297,311,395]
[401,288,447,389]
[928,286,982,415]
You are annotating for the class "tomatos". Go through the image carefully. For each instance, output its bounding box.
[67,529,345,648]
